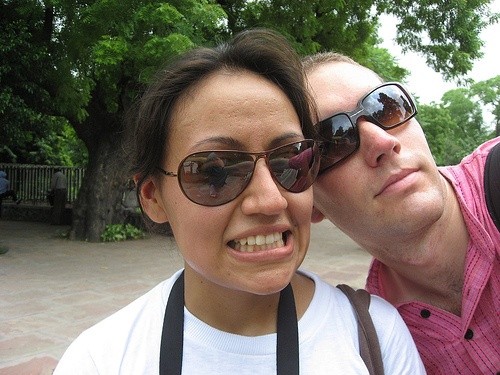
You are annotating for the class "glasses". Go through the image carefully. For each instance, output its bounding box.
[312,81,418,177]
[152,139,321,207]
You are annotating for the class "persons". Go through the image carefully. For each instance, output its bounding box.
[50,166,68,224]
[52,27,427,375]
[300,52,500,375]
[0,171,22,215]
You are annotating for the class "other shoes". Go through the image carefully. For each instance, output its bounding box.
[209,193,216,198]
[16,198,22,204]
